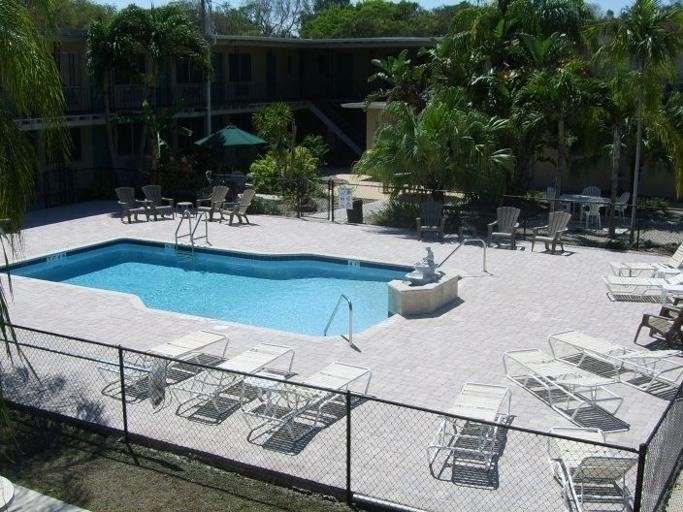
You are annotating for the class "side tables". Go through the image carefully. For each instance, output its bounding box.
[175,201,194,218]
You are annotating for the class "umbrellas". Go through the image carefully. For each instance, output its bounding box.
[193,120,269,168]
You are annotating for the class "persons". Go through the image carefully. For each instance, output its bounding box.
[230,167,244,188]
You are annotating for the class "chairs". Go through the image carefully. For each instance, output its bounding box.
[632,304,683,350]
[101,329,373,440]
[195,185,255,227]
[550,329,682,392]
[426,381,512,477]
[114,183,175,223]
[601,240,682,303]
[415,200,447,245]
[504,348,623,420]
[545,184,630,230]
[485,206,572,254]
[546,426,639,510]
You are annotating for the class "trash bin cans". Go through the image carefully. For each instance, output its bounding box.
[347,199,363,223]
